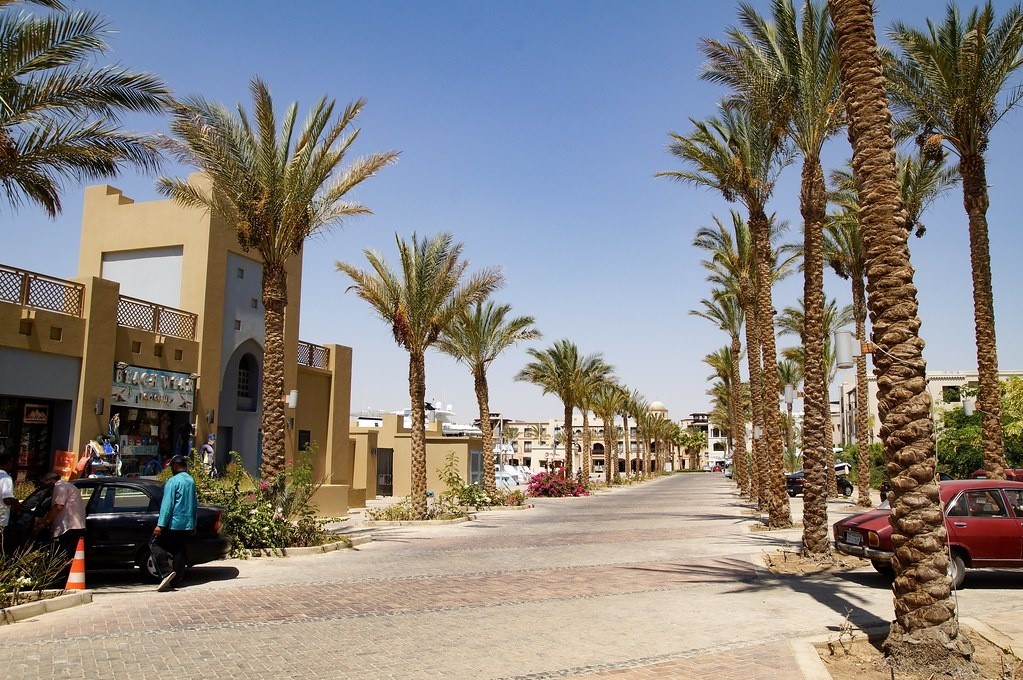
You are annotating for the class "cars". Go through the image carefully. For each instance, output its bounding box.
[1,474,233,584]
[702,465,711,472]
[835,462,854,476]
[785,469,853,497]
[713,465,722,472]
[831,472,1023,590]
[879,472,954,502]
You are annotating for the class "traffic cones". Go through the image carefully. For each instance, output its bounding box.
[64,536,86,589]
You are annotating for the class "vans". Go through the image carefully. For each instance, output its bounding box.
[724,459,733,479]
[966,466,1023,514]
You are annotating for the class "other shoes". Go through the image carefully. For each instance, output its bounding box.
[157,571,176,590]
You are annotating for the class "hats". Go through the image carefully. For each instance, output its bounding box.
[166,455,185,466]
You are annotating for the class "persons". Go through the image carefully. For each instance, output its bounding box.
[36,472,86,589]
[109,413,120,444]
[0,452,30,562]
[577,467,582,483]
[175,432,196,461]
[199,434,217,479]
[150,454,197,592]
